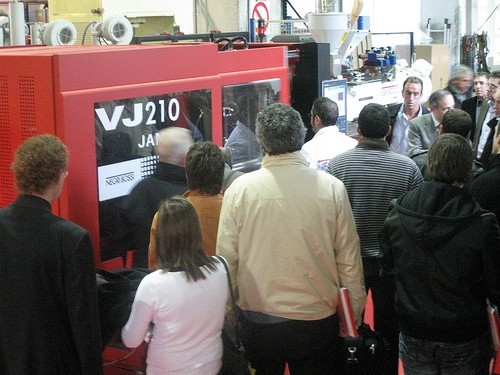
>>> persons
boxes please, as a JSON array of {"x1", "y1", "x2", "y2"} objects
[
  {"x1": 0, "y1": 66, "x2": 500, "y2": 375},
  {"x1": 225, "y1": 93, "x2": 267, "y2": 165}
]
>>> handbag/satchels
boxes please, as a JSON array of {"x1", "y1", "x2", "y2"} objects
[
  {"x1": 345, "y1": 322, "x2": 382, "y2": 375},
  {"x1": 220, "y1": 330, "x2": 250, "y2": 375}
]
